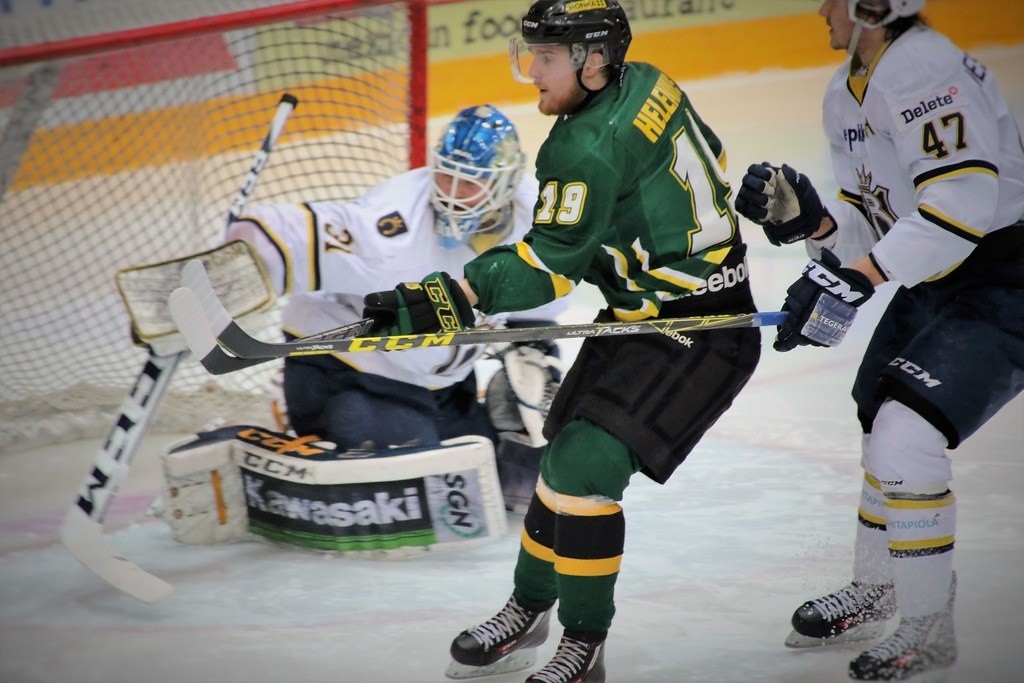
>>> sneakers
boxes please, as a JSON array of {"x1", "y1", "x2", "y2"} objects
[
  {"x1": 848, "y1": 614, "x2": 957, "y2": 682},
  {"x1": 784, "y1": 577, "x2": 896, "y2": 649},
  {"x1": 445, "y1": 594, "x2": 550, "y2": 679},
  {"x1": 523, "y1": 633, "x2": 607, "y2": 683}
]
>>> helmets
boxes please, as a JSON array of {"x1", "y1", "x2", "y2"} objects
[
  {"x1": 509, "y1": 0, "x2": 632, "y2": 83},
  {"x1": 846, "y1": 0, "x2": 925, "y2": 59},
  {"x1": 429, "y1": 103, "x2": 523, "y2": 241}
]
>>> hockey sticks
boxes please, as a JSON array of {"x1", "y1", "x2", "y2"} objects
[
  {"x1": 60, "y1": 92, "x2": 304, "y2": 607},
  {"x1": 161, "y1": 284, "x2": 376, "y2": 379},
  {"x1": 176, "y1": 253, "x2": 790, "y2": 364}
]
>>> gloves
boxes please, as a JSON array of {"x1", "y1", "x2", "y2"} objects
[
  {"x1": 734, "y1": 160, "x2": 837, "y2": 246},
  {"x1": 362, "y1": 271, "x2": 475, "y2": 337},
  {"x1": 773, "y1": 249, "x2": 876, "y2": 353}
]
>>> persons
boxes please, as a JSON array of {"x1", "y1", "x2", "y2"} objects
[
  {"x1": 225, "y1": 1, "x2": 761, "y2": 683},
  {"x1": 736, "y1": 0, "x2": 1024, "y2": 681}
]
